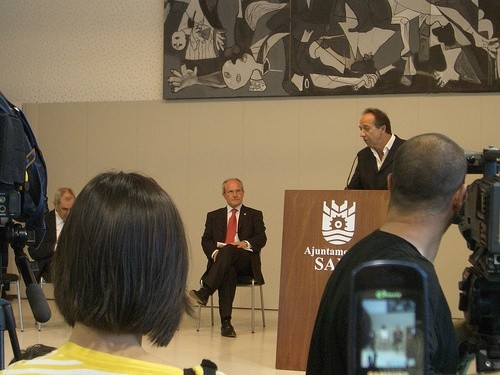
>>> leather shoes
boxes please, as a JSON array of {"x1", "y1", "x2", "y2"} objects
[
  {"x1": 222, "y1": 324, "x2": 236, "y2": 336},
  {"x1": 190, "y1": 288, "x2": 208, "y2": 308}
]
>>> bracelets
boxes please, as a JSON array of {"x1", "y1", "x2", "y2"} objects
[{"x1": 244, "y1": 242, "x2": 248, "y2": 248}]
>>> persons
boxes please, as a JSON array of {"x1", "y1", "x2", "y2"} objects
[
  {"x1": 379, "y1": 321, "x2": 404, "y2": 352},
  {"x1": 344, "y1": 108, "x2": 407, "y2": 191},
  {"x1": 41, "y1": 187, "x2": 76, "y2": 283},
  {"x1": 0, "y1": 168, "x2": 229, "y2": 375},
  {"x1": 189, "y1": 178, "x2": 268, "y2": 337},
  {"x1": 306, "y1": 132, "x2": 478, "y2": 375}
]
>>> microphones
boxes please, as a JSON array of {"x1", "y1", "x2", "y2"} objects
[
  {"x1": 345, "y1": 150, "x2": 366, "y2": 190},
  {"x1": 14, "y1": 246, "x2": 51, "y2": 323}
]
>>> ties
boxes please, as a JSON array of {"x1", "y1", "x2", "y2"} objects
[{"x1": 226, "y1": 208, "x2": 238, "y2": 243}]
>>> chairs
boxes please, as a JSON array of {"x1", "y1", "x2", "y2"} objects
[{"x1": 196, "y1": 271, "x2": 266, "y2": 333}]
